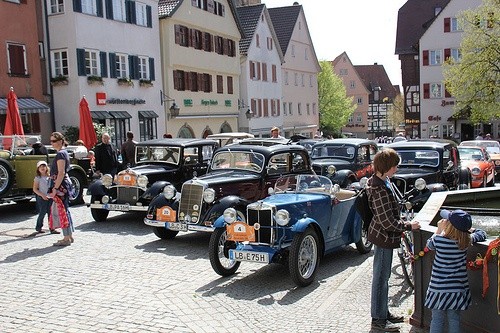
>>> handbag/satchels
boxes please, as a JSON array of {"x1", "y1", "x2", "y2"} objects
[{"x1": 355, "y1": 187, "x2": 373, "y2": 232}]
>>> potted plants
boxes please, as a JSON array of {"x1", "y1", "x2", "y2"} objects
[
  {"x1": 140, "y1": 79, "x2": 154, "y2": 87},
  {"x1": 118, "y1": 78, "x2": 135, "y2": 88},
  {"x1": 88, "y1": 75, "x2": 105, "y2": 86},
  {"x1": 51, "y1": 74, "x2": 69, "y2": 86}
]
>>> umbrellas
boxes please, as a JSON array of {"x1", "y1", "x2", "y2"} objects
[
  {"x1": 4, "y1": 86, "x2": 27, "y2": 149},
  {"x1": 78, "y1": 97, "x2": 97, "y2": 152}
]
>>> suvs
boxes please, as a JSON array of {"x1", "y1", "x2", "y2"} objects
[{"x1": 0, "y1": 132, "x2": 92, "y2": 207}]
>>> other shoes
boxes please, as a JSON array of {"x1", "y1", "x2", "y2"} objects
[
  {"x1": 53, "y1": 238, "x2": 74, "y2": 246},
  {"x1": 386, "y1": 312, "x2": 404, "y2": 322},
  {"x1": 51, "y1": 231, "x2": 60, "y2": 234},
  {"x1": 371, "y1": 317, "x2": 401, "y2": 332},
  {"x1": 39, "y1": 230, "x2": 45, "y2": 233}
]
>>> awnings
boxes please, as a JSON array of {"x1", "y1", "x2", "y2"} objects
[{"x1": 0, "y1": 98, "x2": 51, "y2": 115}]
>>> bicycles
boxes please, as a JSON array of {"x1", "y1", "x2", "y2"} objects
[{"x1": 393, "y1": 177, "x2": 430, "y2": 290}]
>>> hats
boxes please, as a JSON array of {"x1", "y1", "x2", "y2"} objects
[
  {"x1": 37, "y1": 161, "x2": 47, "y2": 168},
  {"x1": 440, "y1": 209, "x2": 472, "y2": 233}
]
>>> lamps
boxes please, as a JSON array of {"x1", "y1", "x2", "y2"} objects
[
  {"x1": 238, "y1": 98, "x2": 251, "y2": 120},
  {"x1": 161, "y1": 89, "x2": 180, "y2": 118}
]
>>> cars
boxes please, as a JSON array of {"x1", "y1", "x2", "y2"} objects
[
  {"x1": 308, "y1": 137, "x2": 380, "y2": 190},
  {"x1": 442, "y1": 145, "x2": 496, "y2": 188},
  {"x1": 458, "y1": 140, "x2": 500, "y2": 182},
  {"x1": 383, "y1": 139, "x2": 473, "y2": 214},
  {"x1": 142, "y1": 139, "x2": 312, "y2": 242},
  {"x1": 207, "y1": 168, "x2": 374, "y2": 288},
  {"x1": 83, "y1": 137, "x2": 220, "y2": 223},
  {"x1": 200, "y1": 133, "x2": 460, "y2": 178}
]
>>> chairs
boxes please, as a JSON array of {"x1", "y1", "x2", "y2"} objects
[
  {"x1": 334, "y1": 148, "x2": 346, "y2": 157},
  {"x1": 472, "y1": 154, "x2": 482, "y2": 160},
  {"x1": 23, "y1": 141, "x2": 46, "y2": 155}
]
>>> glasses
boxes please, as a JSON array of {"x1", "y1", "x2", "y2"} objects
[{"x1": 50, "y1": 138, "x2": 62, "y2": 143}]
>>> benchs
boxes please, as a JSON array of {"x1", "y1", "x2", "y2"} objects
[{"x1": 301, "y1": 185, "x2": 357, "y2": 200}]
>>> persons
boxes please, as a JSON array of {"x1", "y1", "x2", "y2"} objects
[
  {"x1": 49, "y1": 131, "x2": 75, "y2": 247},
  {"x1": 365, "y1": 146, "x2": 420, "y2": 332},
  {"x1": 474, "y1": 131, "x2": 492, "y2": 140},
  {"x1": 32, "y1": 160, "x2": 60, "y2": 234},
  {"x1": 424, "y1": 208, "x2": 487, "y2": 333},
  {"x1": 121, "y1": 131, "x2": 140, "y2": 168},
  {"x1": 270, "y1": 126, "x2": 286, "y2": 139},
  {"x1": 92, "y1": 133, "x2": 115, "y2": 180}
]
